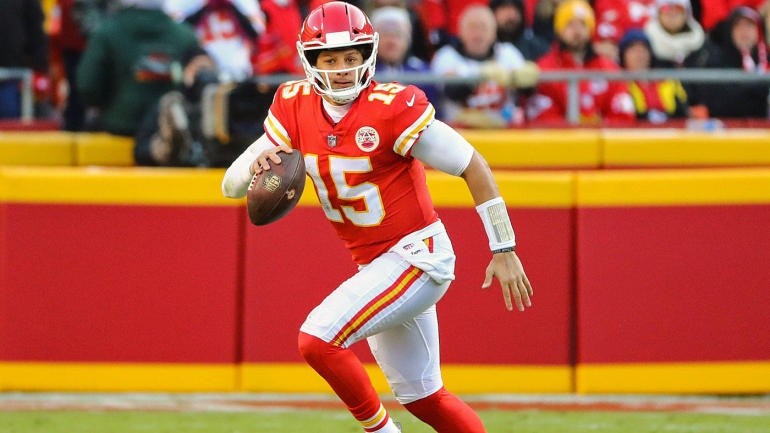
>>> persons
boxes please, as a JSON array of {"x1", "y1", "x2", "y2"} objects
[
  {"x1": 0, "y1": 0, "x2": 769, "y2": 171},
  {"x1": 220, "y1": 0, "x2": 536, "y2": 433}
]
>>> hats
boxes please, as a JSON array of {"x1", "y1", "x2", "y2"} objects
[
  {"x1": 554, "y1": 1, "x2": 596, "y2": 36},
  {"x1": 655, "y1": 0, "x2": 691, "y2": 21},
  {"x1": 619, "y1": 28, "x2": 652, "y2": 55},
  {"x1": 729, "y1": 6, "x2": 761, "y2": 24}
]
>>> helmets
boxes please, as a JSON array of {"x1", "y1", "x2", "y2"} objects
[{"x1": 297, "y1": 1, "x2": 378, "y2": 105}]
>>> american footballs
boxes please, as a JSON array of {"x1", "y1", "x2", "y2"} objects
[{"x1": 247, "y1": 148, "x2": 306, "y2": 225}]
